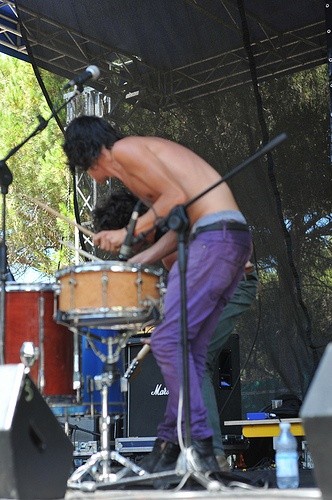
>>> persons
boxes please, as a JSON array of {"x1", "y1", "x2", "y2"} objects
[
  {"x1": 139, "y1": 211, "x2": 258, "y2": 470},
  {"x1": 63, "y1": 116, "x2": 253, "y2": 474}
]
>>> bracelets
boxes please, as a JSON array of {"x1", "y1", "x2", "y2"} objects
[{"x1": 125, "y1": 225, "x2": 136, "y2": 238}]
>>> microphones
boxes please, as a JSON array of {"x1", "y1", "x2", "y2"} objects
[
  {"x1": 119, "y1": 203, "x2": 141, "y2": 262},
  {"x1": 62, "y1": 65, "x2": 100, "y2": 90}
]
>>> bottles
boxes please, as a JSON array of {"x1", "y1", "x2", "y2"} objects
[{"x1": 275, "y1": 423, "x2": 298, "y2": 489}]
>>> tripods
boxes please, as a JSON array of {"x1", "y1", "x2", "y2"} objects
[{"x1": 67, "y1": 131, "x2": 288, "y2": 492}]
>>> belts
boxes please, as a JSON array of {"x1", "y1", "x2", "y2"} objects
[
  {"x1": 191, "y1": 223, "x2": 250, "y2": 242},
  {"x1": 240, "y1": 274, "x2": 258, "y2": 282}
]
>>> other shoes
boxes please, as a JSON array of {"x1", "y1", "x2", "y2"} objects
[{"x1": 98, "y1": 436, "x2": 221, "y2": 490}]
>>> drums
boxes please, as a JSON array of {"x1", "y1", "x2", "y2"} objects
[
  {"x1": 0, "y1": 280, "x2": 85, "y2": 409},
  {"x1": 52, "y1": 261, "x2": 163, "y2": 328}
]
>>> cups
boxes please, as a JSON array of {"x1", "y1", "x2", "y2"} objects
[{"x1": 272, "y1": 400, "x2": 282, "y2": 409}]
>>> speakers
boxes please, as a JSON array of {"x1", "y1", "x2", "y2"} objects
[
  {"x1": 297, "y1": 342, "x2": 332, "y2": 500},
  {"x1": 125, "y1": 333, "x2": 243, "y2": 439},
  {"x1": 0, "y1": 361, "x2": 76, "y2": 500}
]
